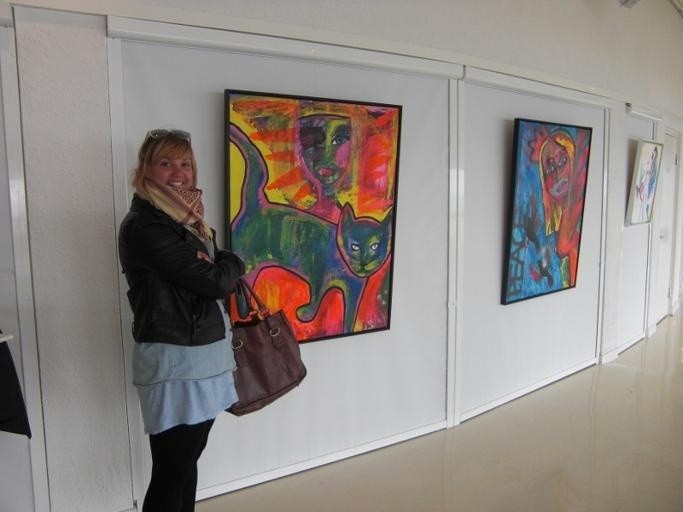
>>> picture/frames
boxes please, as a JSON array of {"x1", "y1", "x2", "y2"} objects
[
  {"x1": 621, "y1": 138, "x2": 664, "y2": 226},
  {"x1": 497, "y1": 114, "x2": 595, "y2": 306},
  {"x1": 220, "y1": 86, "x2": 405, "y2": 344}
]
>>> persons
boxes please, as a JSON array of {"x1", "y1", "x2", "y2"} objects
[
  {"x1": 540, "y1": 129, "x2": 588, "y2": 288},
  {"x1": 118, "y1": 129, "x2": 245, "y2": 512},
  {"x1": 240, "y1": 97, "x2": 397, "y2": 342},
  {"x1": 637, "y1": 146, "x2": 658, "y2": 222}
]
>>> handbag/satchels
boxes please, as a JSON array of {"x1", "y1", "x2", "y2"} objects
[{"x1": 223, "y1": 309, "x2": 306, "y2": 416}]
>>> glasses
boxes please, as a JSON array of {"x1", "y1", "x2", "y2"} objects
[{"x1": 143, "y1": 129, "x2": 192, "y2": 146}]
